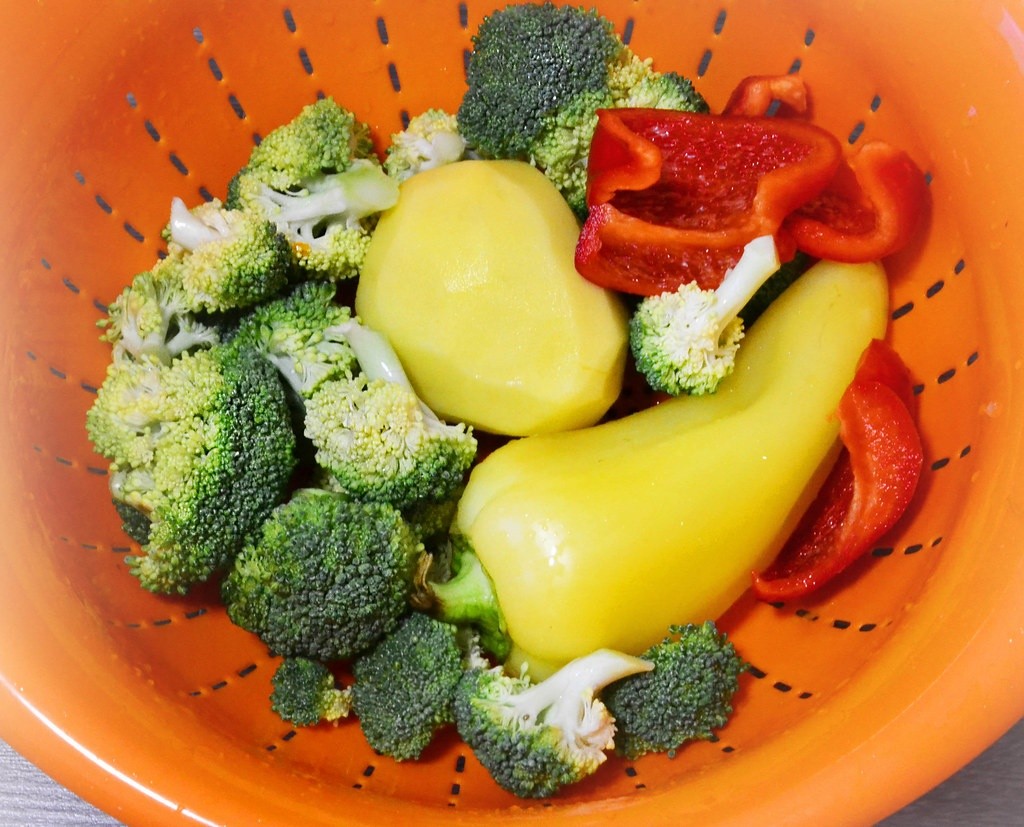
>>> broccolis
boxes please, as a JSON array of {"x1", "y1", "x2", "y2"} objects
[{"x1": 85, "y1": 2, "x2": 780, "y2": 801}]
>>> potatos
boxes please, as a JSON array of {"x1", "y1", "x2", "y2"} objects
[{"x1": 351, "y1": 160, "x2": 627, "y2": 437}]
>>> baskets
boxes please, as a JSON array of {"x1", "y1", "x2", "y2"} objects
[{"x1": 0, "y1": 0, "x2": 1024, "y2": 827}]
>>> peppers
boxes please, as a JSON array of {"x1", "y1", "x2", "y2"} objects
[{"x1": 419, "y1": 70, "x2": 927, "y2": 687}]
